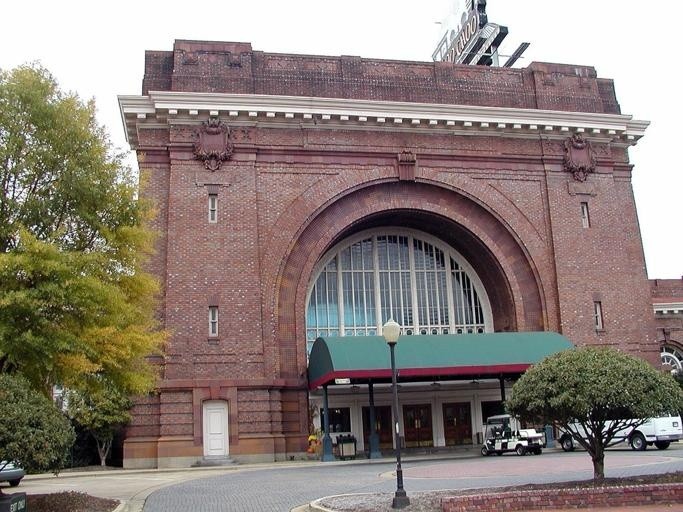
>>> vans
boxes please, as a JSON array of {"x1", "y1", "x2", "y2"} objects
[{"x1": 558, "y1": 404, "x2": 683, "y2": 452}]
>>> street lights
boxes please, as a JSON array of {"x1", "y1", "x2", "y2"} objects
[{"x1": 380, "y1": 316, "x2": 411, "y2": 508}]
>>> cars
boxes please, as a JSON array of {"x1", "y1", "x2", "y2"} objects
[{"x1": 0, "y1": 455, "x2": 24, "y2": 489}]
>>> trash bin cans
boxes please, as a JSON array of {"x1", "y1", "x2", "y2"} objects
[{"x1": 336, "y1": 434, "x2": 357, "y2": 460}]
